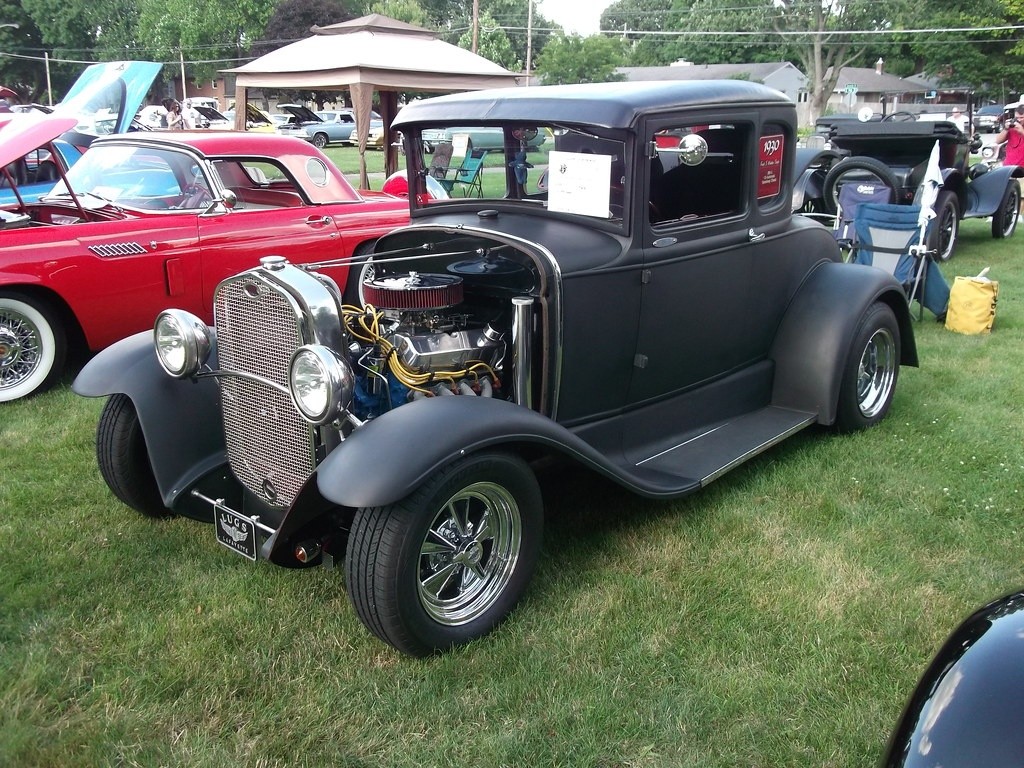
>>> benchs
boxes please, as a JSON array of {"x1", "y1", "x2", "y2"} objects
[
  {"x1": 829, "y1": 119, "x2": 969, "y2": 169},
  {"x1": 186, "y1": 186, "x2": 301, "y2": 218}
]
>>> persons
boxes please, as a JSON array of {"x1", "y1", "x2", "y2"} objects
[
  {"x1": 994, "y1": 104, "x2": 1024, "y2": 222},
  {"x1": 945, "y1": 107, "x2": 975, "y2": 136},
  {"x1": 165, "y1": 101, "x2": 183, "y2": 130}
]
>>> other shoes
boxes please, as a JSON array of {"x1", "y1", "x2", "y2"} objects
[
  {"x1": 1017, "y1": 214, "x2": 1023, "y2": 223},
  {"x1": 986, "y1": 216, "x2": 993, "y2": 223}
]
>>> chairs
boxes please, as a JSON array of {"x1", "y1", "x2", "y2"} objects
[
  {"x1": 562, "y1": 131, "x2": 664, "y2": 200},
  {"x1": 652, "y1": 128, "x2": 749, "y2": 220},
  {"x1": 839, "y1": 202, "x2": 933, "y2": 324},
  {"x1": 433, "y1": 148, "x2": 488, "y2": 199},
  {"x1": 426, "y1": 142, "x2": 454, "y2": 181},
  {"x1": 34, "y1": 160, "x2": 58, "y2": 181},
  {"x1": 796, "y1": 183, "x2": 891, "y2": 264},
  {"x1": 0, "y1": 156, "x2": 29, "y2": 188}
]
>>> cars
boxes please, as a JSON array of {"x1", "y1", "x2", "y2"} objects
[
  {"x1": 0, "y1": 133, "x2": 450, "y2": 406},
  {"x1": 347, "y1": 119, "x2": 384, "y2": 150},
  {"x1": 275, "y1": 104, "x2": 357, "y2": 149},
  {"x1": 789, "y1": 90, "x2": 1024, "y2": 263},
  {"x1": 0, "y1": 62, "x2": 264, "y2": 209},
  {"x1": 71, "y1": 80, "x2": 921, "y2": 658}
]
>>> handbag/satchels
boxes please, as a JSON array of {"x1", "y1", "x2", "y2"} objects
[{"x1": 944, "y1": 276, "x2": 999, "y2": 336}]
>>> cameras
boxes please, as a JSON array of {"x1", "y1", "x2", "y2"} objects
[{"x1": 1008, "y1": 118, "x2": 1016, "y2": 128}]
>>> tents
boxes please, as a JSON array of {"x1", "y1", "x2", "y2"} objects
[{"x1": 215, "y1": 12, "x2": 535, "y2": 200}]
